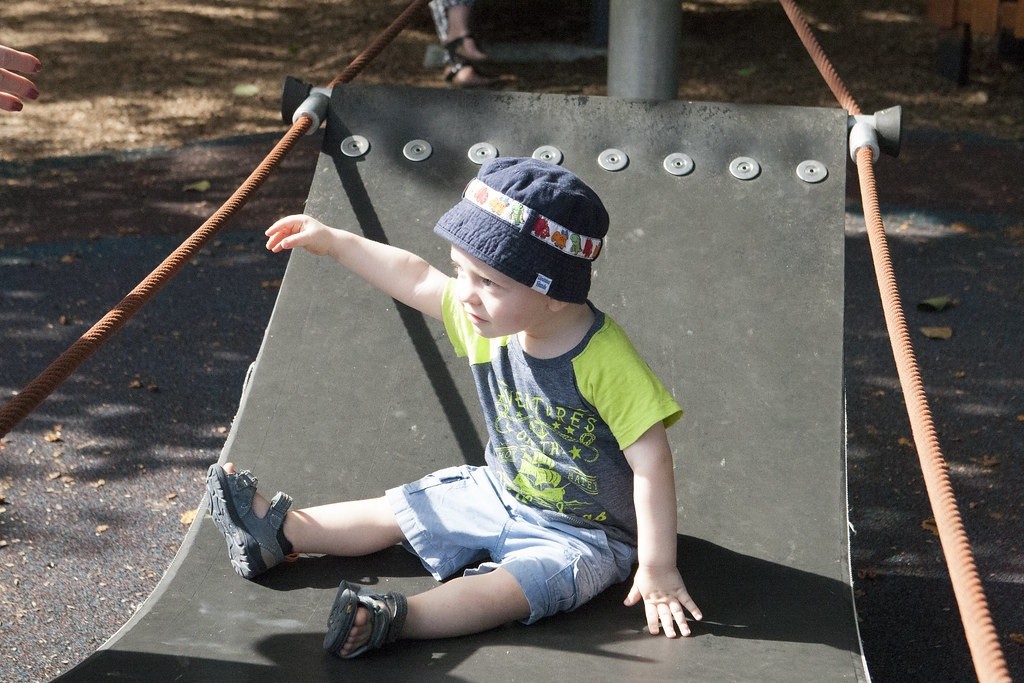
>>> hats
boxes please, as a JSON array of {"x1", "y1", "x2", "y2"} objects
[{"x1": 434, "y1": 156, "x2": 609, "y2": 306}]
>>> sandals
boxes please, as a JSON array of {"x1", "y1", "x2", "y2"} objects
[
  {"x1": 208, "y1": 463, "x2": 293, "y2": 578},
  {"x1": 323, "y1": 580, "x2": 408, "y2": 658}
]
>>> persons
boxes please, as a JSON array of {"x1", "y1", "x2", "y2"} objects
[{"x1": 206, "y1": 156, "x2": 703, "y2": 661}]
